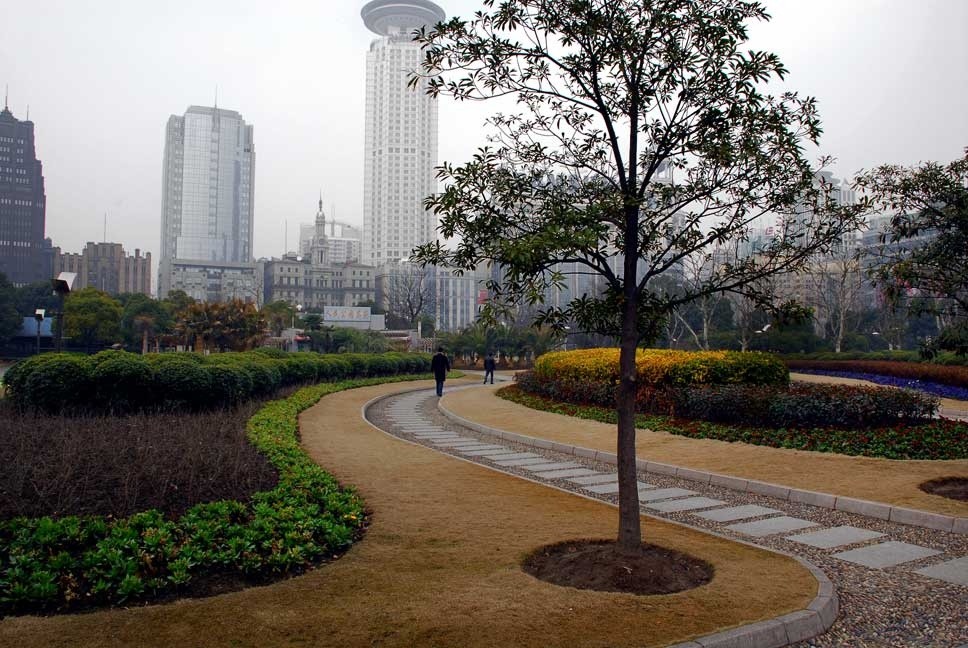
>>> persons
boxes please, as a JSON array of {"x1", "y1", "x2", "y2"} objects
[
  {"x1": 431, "y1": 347, "x2": 451, "y2": 396},
  {"x1": 484, "y1": 352, "x2": 496, "y2": 384}
]
